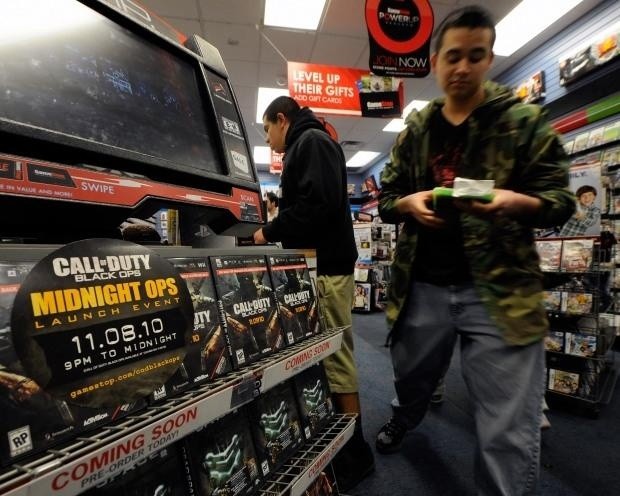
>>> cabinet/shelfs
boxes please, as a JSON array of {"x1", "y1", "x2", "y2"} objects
[
  {"x1": 1, "y1": 243, "x2": 359, "y2": 496},
  {"x1": 351, "y1": 91, "x2": 620, "y2": 419}
]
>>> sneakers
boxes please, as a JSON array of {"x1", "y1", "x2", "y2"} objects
[
  {"x1": 337, "y1": 442, "x2": 375, "y2": 493},
  {"x1": 430, "y1": 383, "x2": 446, "y2": 403},
  {"x1": 375, "y1": 418, "x2": 406, "y2": 455}
]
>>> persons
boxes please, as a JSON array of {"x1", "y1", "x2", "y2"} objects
[
  {"x1": 378, "y1": 6, "x2": 575, "y2": 453},
  {"x1": 254, "y1": 96, "x2": 372, "y2": 454},
  {"x1": 264, "y1": 192, "x2": 279, "y2": 222},
  {"x1": 561, "y1": 185, "x2": 601, "y2": 236}
]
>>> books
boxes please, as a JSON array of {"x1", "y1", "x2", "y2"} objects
[
  {"x1": 0, "y1": 255, "x2": 333, "y2": 496},
  {"x1": 535, "y1": 240, "x2": 619, "y2": 394},
  {"x1": 562, "y1": 122, "x2": 620, "y2": 239}
]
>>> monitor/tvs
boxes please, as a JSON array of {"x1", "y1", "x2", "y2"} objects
[{"x1": 0, "y1": 0, "x2": 228, "y2": 176}]
